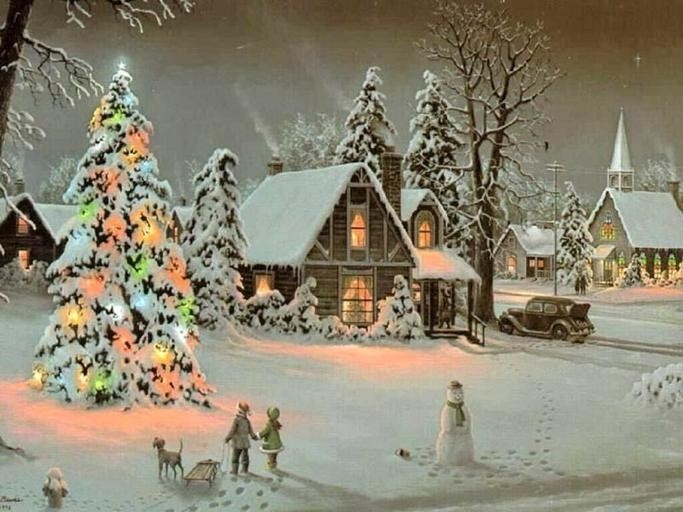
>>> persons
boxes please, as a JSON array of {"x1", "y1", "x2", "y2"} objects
[
  {"x1": 223, "y1": 400, "x2": 257, "y2": 474},
  {"x1": 437, "y1": 287, "x2": 452, "y2": 329},
  {"x1": 574, "y1": 276, "x2": 581, "y2": 295},
  {"x1": 579, "y1": 274, "x2": 588, "y2": 296},
  {"x1": 256, "y1": 404, "x2": 284, "y2": 473}
]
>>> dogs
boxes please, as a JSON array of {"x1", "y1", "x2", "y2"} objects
[
  {"x1": 151, "y1": 435, "x2": 184, "y2": 482},
  {"x1": 41, "y1": 465, "x2": 66, "y2": 508}
]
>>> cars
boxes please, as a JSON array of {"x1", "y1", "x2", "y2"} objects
[{"x1": 496, "y1": 296, "x2": 594, "y2": 342}]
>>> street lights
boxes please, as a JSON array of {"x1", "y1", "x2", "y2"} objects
[{"x1": 543, "y1": 160, "x2": 565, "y2": 296}]
